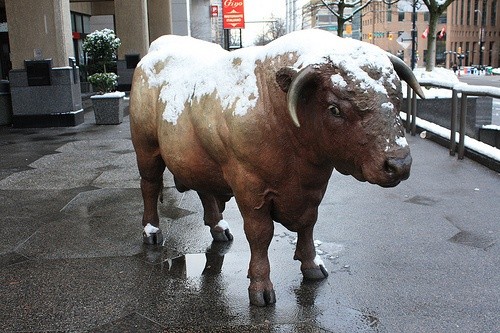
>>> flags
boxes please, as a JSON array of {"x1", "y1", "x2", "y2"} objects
[
  {"x1": 422, "y1": 26, "x2": 429, "y2": 38},
  {"x1": 439, "y1": 27, "x2": 446, "y2": 39}
]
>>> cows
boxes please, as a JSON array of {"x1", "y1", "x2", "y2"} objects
[{"x1": 129, "y1": 28, "x2": 427, "y2": 307}]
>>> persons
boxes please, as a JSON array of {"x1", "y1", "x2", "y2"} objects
[{"x1": 453, "y1": 63, "x2": 459, "y2": 74}]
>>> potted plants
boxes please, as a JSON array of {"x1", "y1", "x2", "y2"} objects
[{"x1": 81, "y1": 27, "x2": 125, "y2": 126}]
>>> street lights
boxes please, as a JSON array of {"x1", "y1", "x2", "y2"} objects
[
  {"x1": 457, "y1": 54, "x2": 465, "y2": 66},
  {"x1": 474, "y1": 10, "x2": 483, "y2": 67}
]
